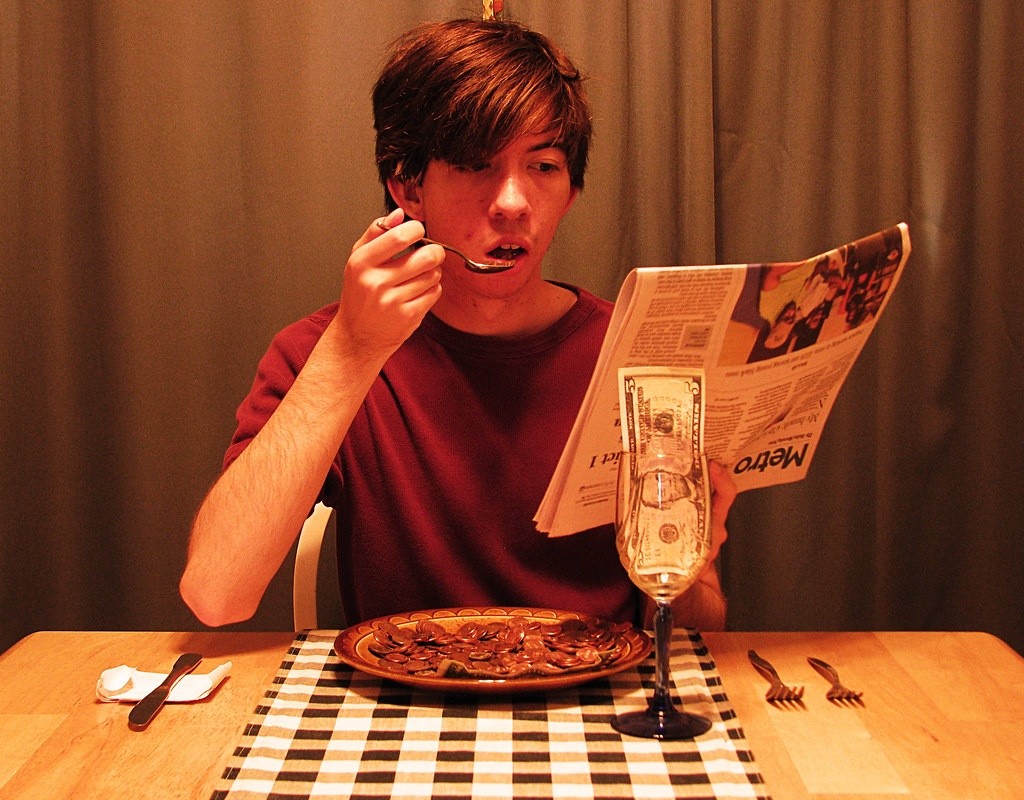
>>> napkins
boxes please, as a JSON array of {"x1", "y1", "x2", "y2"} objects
[{"x1": 96, "y1": 664, "x2": 231, "y2": 702}]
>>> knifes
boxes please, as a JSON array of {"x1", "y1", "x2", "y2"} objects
[{"x1": 128, "y1": 653, "x2": 202, "y2": 727}]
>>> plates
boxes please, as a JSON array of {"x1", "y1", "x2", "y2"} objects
[{"x1": 333, "y1": 605, "x2": 653, "y2": 703}]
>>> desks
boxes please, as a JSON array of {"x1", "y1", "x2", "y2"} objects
[{"x1": 0, "y1": 632, "x2": 1024, "y2": 800}]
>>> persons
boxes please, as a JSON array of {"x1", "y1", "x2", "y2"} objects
[
  {"x1": 757, "y1": 245, "x2": 832, "y2": 347},
  {"x1": 179, "y1": 16, "x2": 739, "y2": 636}
]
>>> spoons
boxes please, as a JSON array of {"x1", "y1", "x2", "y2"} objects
[{"x1": 375, "y1": 222, "x2": 517, "y2": 274}]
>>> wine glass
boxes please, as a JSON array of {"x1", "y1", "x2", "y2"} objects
[{"x1": 609, "y1": 448, "x2": 714, "y2": 740}]
